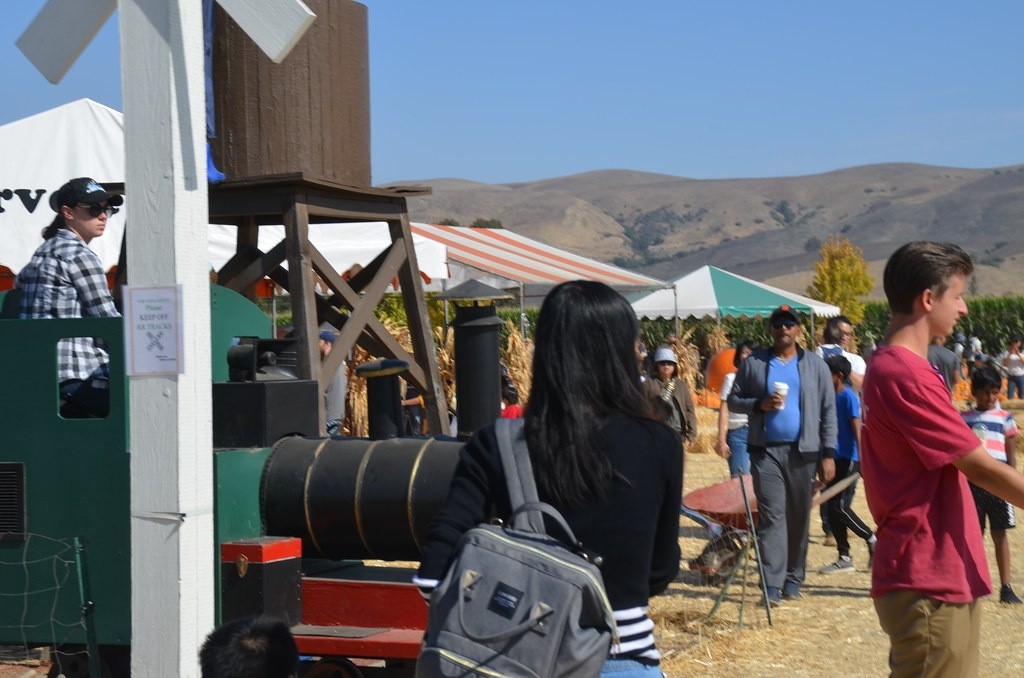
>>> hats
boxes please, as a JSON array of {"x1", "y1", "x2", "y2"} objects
[
  {"x1": 654, "y1": 348, "x2": 677, "y2": 365},
  {"x1": 57, "y1": 177, "x2": 123, "y2": 210},
  {"x1": 770, "y1": 305, "x2": 801, "y2": 323},
  {"x1": 321, "y1": 330, "x2": 336, "y2": 343}
]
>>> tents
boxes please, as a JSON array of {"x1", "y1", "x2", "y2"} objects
[
  {"x1": 0, "y1": 97, "x2": 678, "y2": 349},
  {"x1": 631, "y1": 266, "x2": 841, "y2": 351}
]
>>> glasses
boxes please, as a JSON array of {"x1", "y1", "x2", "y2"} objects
[
  {"x1": 70, "y1": 202, "x2": 113, "y2": 218},
  {"x1": 770, "y1": 318, "x2": 797, "y2": 328}
]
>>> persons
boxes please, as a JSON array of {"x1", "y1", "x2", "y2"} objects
[
  {"x1": 318, "y1": 332, "x2": 346, "y2": 437},
  {"x1": 13, "y1": 178, "x2": 123, "y2": 414},
  {"x1": 198, "y1": 615, "x2": 303, "y2": 678},
  {"x1": 415, "y1": 241, "x2": 1024, "y2": 678}
]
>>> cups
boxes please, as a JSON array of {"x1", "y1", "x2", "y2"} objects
[
  {"x1": 972, "y1": 423, "x2": 988, "y2": 451},
  {"x1": 772, "y1": 382, "x2": 790, "y2": 410}
]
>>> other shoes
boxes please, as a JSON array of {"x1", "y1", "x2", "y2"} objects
[
  {"x1": 820, "y1": 556, "x2": 854, "y2": 574},
  {"x1": 1000, "y1": 587, "x2": 1021, "y2": 603},
  {"x1": 826, "y1": 535, "x2": 836, "y2": 546},
  {"x1": 867, "y1": 540, "x2": 877, "y2": 565}
]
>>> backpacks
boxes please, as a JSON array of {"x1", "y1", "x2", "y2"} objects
[{"x1": 413, "y1": 418, "x2": 619, "y2": 678}]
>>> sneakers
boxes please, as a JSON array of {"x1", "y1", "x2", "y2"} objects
[
  {"x1": 761, "y1": 587, "x2": 780, "y2": 607},
  {"x1": 783, "y1": 579, "x2": 801, "y2": 600}
]
